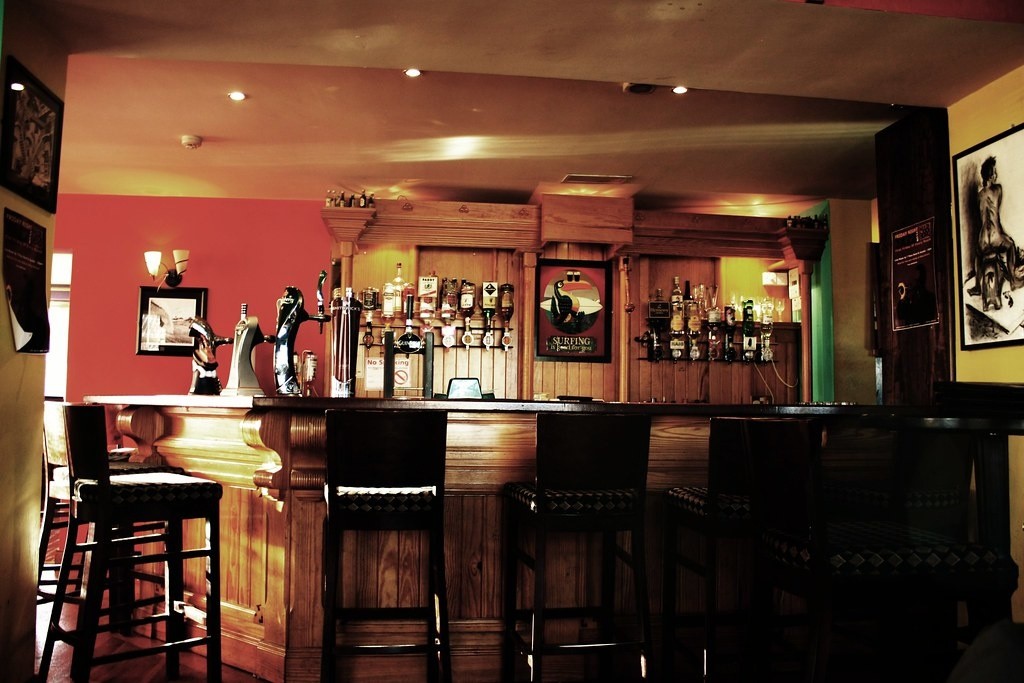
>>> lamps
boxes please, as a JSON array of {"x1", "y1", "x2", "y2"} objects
[{"x1": 143, "y1": 249, "x2": 190, "y2": 288}]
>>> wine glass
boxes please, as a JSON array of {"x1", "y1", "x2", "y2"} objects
[{"x1": 729, "y1": 293, "x2": 785, "y2": 322}]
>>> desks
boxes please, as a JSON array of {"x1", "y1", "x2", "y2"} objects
[{"x1": 84, "y1": 393, "x2": 1015, "y2": 681}]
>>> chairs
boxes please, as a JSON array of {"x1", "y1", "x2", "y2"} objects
[
  {"x1": 658, "y1": 415, "x2": 817, "y2": 683},
  {"x1": 498, "y1": 412, "x2": 653, "y2": 683},
  {"x1": 39, "y1": 399, "x2": 188, "y2": 653},
  {"x1": 37, "y1": 404, "x2": 223, "y2": 683},
  {"x1": 744, "y1": 419, "x2": 1018, "y2": 682},
  {"x1": 319, "y1": 407, "x2": 452, "y2": 682}
]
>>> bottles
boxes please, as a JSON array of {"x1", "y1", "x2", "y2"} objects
[
  {"x1": 236, "y1": 302, "x2": 248, "y2": 335},
  {"x1": 294, "y1": 352, "x2": 318, "y2": 397},
  {"x1": 784, "y1": 215, "x2": 828, "y2": 230},
  {"x1": 326, "y1": 189, "x2": 375, "y2": 208},
  {"x1": 333, "y1": 262, "x2": 515, "y2": 352},
  {"x1": 646, "y1": 276, "x2": 774, "y2": 365}
]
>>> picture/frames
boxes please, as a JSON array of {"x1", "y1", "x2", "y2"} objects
[
  {"x1": 0, "y1": 53, "x2": 64, "y2": 215},
  {"x1": 952, "y1": 122, "x2": 1024, "y2": 352},
  {"x1": 135, "y1": 285, "x2": 209, "y2": 357}
]
